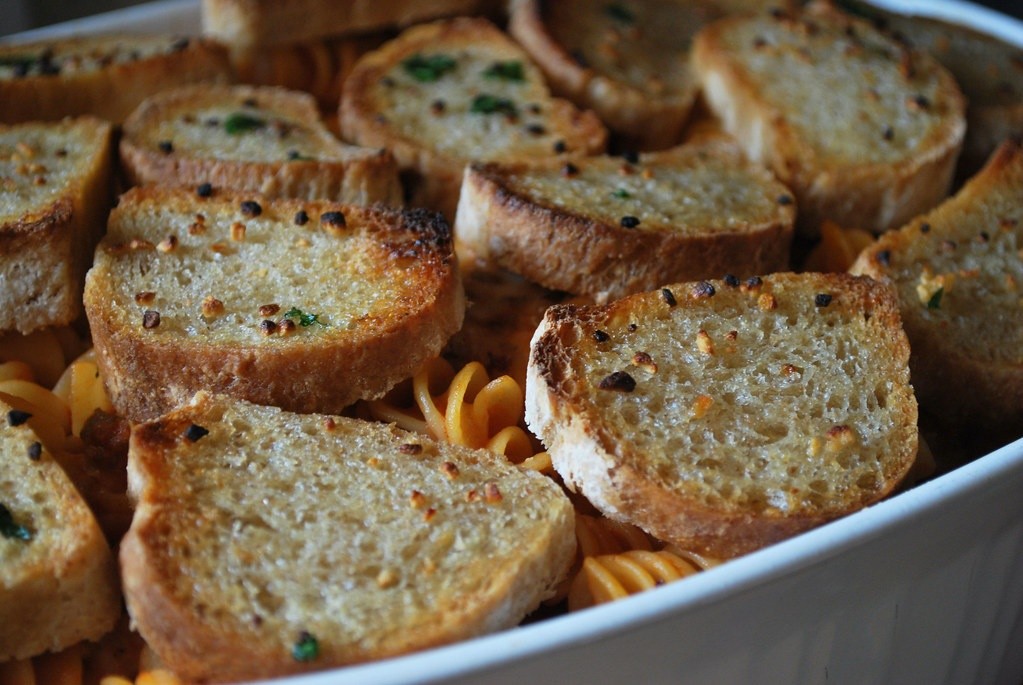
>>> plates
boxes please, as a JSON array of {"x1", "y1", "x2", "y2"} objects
[{"x1": 0, "y1": 0, "x2": 1023, "y2": 685}]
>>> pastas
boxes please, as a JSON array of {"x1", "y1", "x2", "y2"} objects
[{"x1": 0, "y1": 219, "x2": 957, "y2": 685}]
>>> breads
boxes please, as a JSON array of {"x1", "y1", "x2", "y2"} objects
[{"x1": 0, "y1": 0, "x2": 1023, "y2": 680}]
